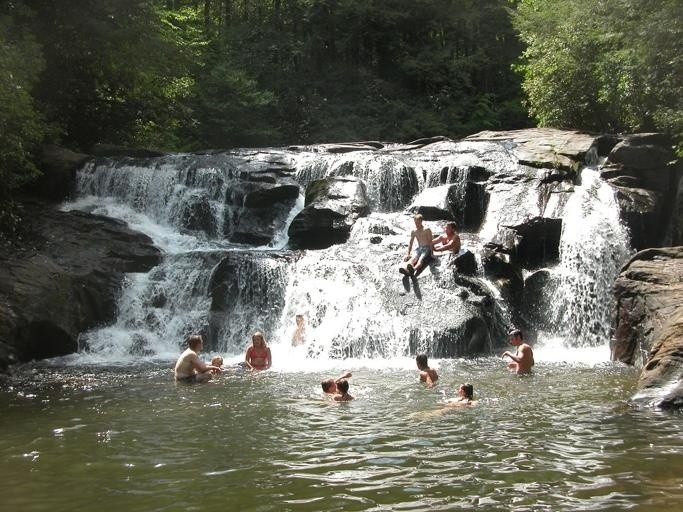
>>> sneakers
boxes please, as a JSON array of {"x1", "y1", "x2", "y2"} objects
[
  {"x1": 407, "y1": 264, "x2": 414, "y2": 274},
  {"x1": 399, "y1": 268, "x2": 409, "y2": 276}
]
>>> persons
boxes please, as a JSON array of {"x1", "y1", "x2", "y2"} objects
[
  {"x1": 501, "y1": 329, "x2": 534, "y2": 375},
  {"x1": 398, "y1": 213, "x2": 432, "y2": 277},
  {"x1": 320, "y1": 370, "x2": 354, "y2": 396},
  {"x1": 291, "y1": 314, "x2": 306, "y2": 346},
  {"x1": 431, "y1": 221, "x2": 460, "y2": 252},
  {"x1": 415, "y1": 355, "x2": 438, "y2": 383},
  {"x1": 173, "y1": 334, "x2": 222, "y2": 384},
  {"x1": 210, "y1": 355, "x2": 225, "y2": 371},
  {"x1": 406, "y1": 383, "x2": 477, "y2": 423},
  {"x1": 333, "y1": 378, "x2": 353, "y2": 401},
  {"x1": 245, "y1": 332, "x2": 271, "y2": 372}
]
